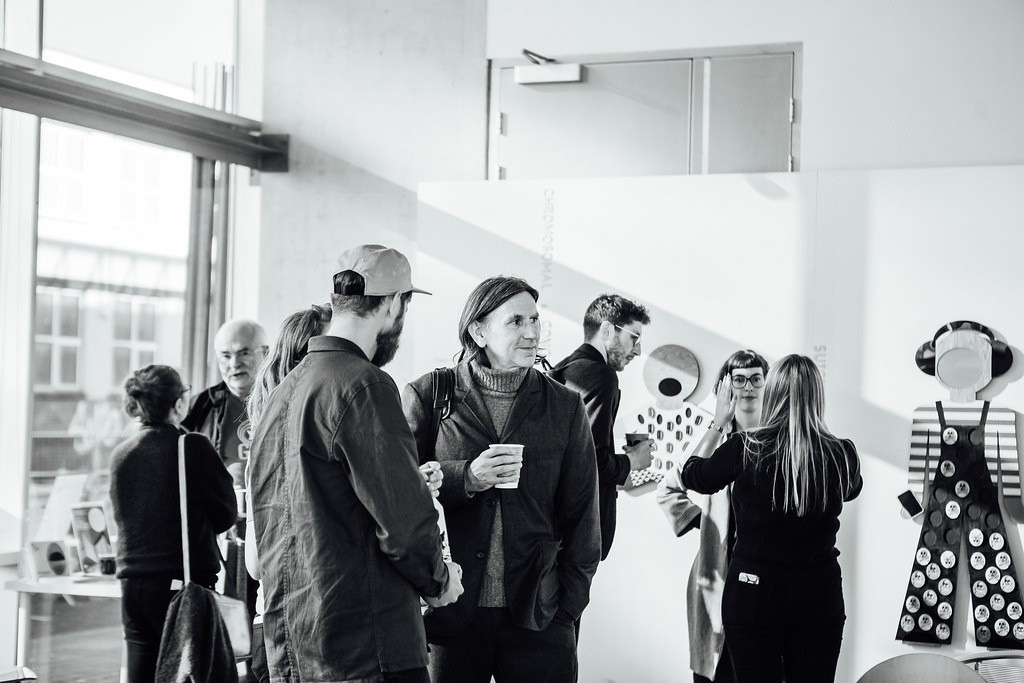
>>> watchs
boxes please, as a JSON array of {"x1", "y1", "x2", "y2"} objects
[{"x1": 708, "y1": 420, "x2": 723, "y2": 434}]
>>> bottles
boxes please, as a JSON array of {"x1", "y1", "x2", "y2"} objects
[{"x1": 423, "y1": 468, "x2": 452, "y2": 562}]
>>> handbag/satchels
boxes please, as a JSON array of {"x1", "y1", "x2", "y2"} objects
[{"x1": 185, "y1": 583, "x2": 253, "y2": 683}]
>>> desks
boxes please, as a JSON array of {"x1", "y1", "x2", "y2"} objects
[{"x1": 3, "y1": 576, "x2": 128, "y2": 683}]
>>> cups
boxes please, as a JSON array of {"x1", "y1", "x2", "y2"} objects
[
  {"x1": 626, "y1": 434, "x2": 649, "y2": 471},
  {"x1": 489, "y1": 444, "x2": 524, "y2": 489},
  {"x1": 98, "y1": 554, "x2": 116, "y2": 574},
  {"x1": 235, "y1": 490, "x2": 246, "y2": 518}
]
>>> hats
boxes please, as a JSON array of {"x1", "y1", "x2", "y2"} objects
[{"x1": 331, "y1": 244, "x2": 433, "y2": 296}]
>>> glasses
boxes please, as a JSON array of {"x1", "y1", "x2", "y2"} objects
[
  {"x1": 172, "y1": 384, "x2": 192, "y2": 408},
  {"x1": 614, "y1": 325, "x2": 641, "y2": 347},
  {"x1": 217, "y1": 349, "x2": 263, "y2": 364},
  {"x1": 731, "y1": 373, "x2": 765, "y2": 389}
]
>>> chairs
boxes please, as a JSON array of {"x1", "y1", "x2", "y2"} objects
[
  {"x1": 857, "y1": 653, "x2": 987, "y2": 683},
  {"x1": 962, "y1": 654, "x2": 1024, "y2": 683}
]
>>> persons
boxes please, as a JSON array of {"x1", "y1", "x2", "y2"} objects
[
  {"x1": 657, "y1": 349, "x2": 864, "y2": 683},
  {"x1": 400, "y1": 277, "x2": 655, "y2": 682},
  {"x1": 109, "y1": 245, "x2": 465, "y2": 683}
]
